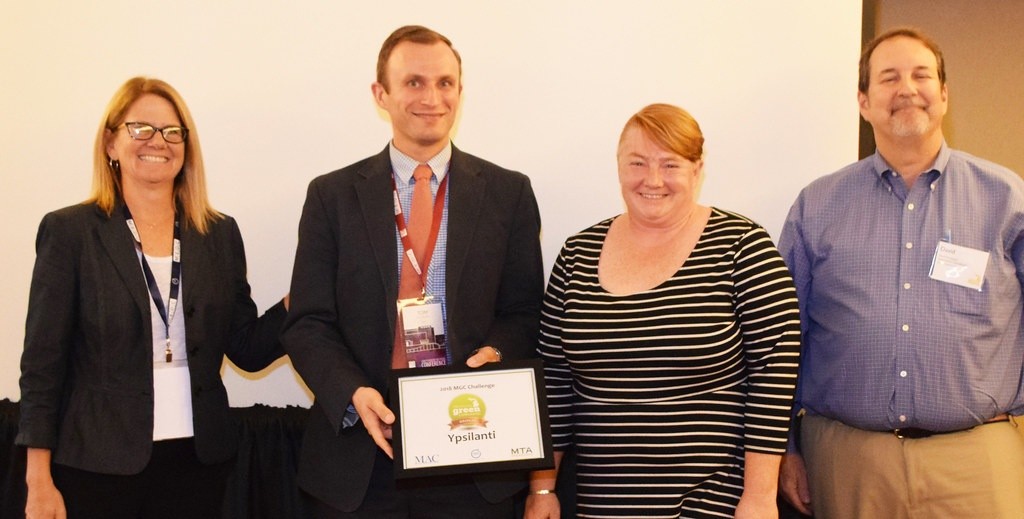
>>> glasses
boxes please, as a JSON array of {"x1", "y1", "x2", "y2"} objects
[{"x1": 112, "y1": 121, "x2": 189, "y2": 144}]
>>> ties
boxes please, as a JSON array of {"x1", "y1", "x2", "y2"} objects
[{"x1": 391, "y1": 164, "x2": 434, "y2": 369}]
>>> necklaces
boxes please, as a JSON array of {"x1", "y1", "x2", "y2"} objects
[{"x1": 142, "y1": 218, "x2": 161, "y2": 230}]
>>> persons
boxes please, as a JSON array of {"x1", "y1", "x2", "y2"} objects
[
  {"x1": 776, "y1": 27, "x2": 1024, "y2": 519},
  {"x1": 16, "y1": 77, "x2": 289, "y2": 519},
  {"x1": 279, "y1": 25, "x2": 543, "y2": 519},
  {"x1": 523, "y1": 103, "x2": 802, "y2": 519}
]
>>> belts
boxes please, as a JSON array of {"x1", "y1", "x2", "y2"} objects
[{"x1": 871, "y1": 414, "x2": 1010, "y2": 441}]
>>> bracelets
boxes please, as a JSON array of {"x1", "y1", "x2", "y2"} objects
[{"x1": 527, "y1": 489, "x2": 555, "y2": 497}]
